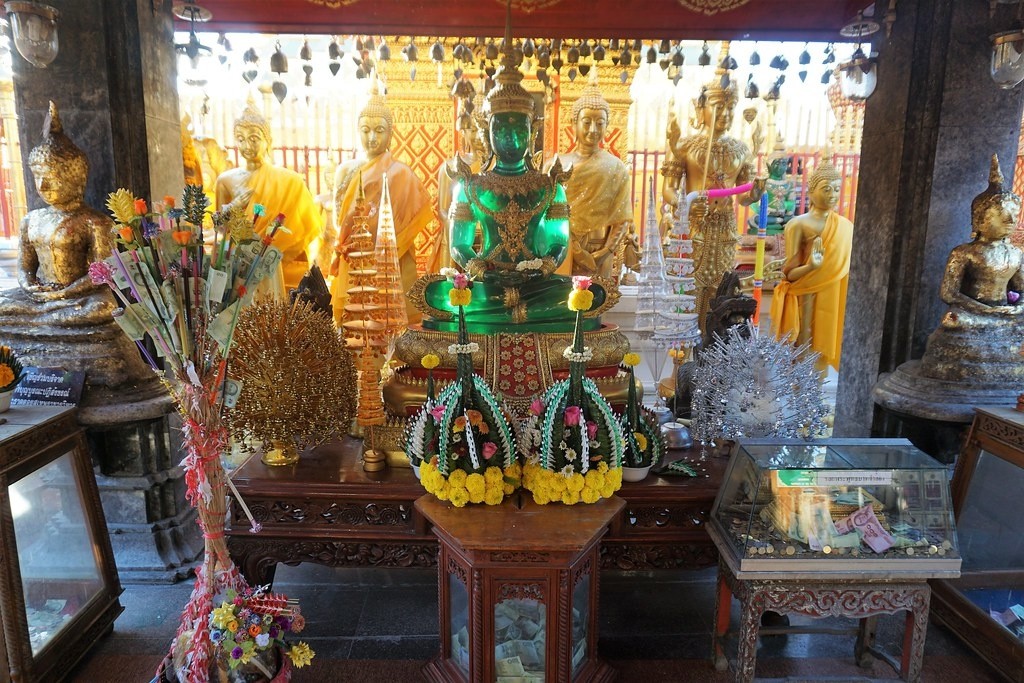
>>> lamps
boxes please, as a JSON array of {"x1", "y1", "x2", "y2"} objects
[
  {"x1": 988, "y1": 29, "x2": 1024, "y2": 90},
  {"x1": 2, "y1": 0, "x2": 61, "y2": 71},
  {"x1": 837, "y1": 10, "x2": 881, "y2": 101},
  {"x1": 171, "y1": 0, "x2": 214, "y2": 69}
]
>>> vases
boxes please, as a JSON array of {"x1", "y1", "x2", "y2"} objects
[
  {"x1": 155, "y1": 642, "x2": 294, "y2": 683},
  {"x1": 0, "y1": 389, "x2": 15, "y2": 413},
  {"x1": 621, "y1": 465, "x2": 652, "y2": 482}
]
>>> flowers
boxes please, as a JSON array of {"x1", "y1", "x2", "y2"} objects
[
  {"x1": 92, "y1": 184, "x2": 315, "y2": 683},
  {"x1": 615, "y1": 352, "x2": 660, "y2": 467},
  {"x1": 0, "y1": 345, "x2": 28, "y2": 393}
]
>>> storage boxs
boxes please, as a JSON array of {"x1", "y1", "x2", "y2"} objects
[
  {"x1": 925, "y1": 407, "x2": 1024, "y2": 683},
  {"x1": 703, "y1": 437, "x2": 962, "y2": 577},
  {"x1": 0, "y1": 403, "x2": 126, "y2": 683}
]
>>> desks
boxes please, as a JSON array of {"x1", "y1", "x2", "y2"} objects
[{"x1": 229, "y1": 430, "x2": 794, "y2": 643}]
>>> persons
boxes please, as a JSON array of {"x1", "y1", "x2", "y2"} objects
[
  {"x1": 541, "y1": 59, "x2": 634, "y2": 284},
  {"x1": 406, "y1": 0, "x2": 606, "y2": 333},
  {"x1": 624, "y1": 223, "x2": 641, "y2": 270},
  {"x1": 747, "y1": 129, "x2": 798, "y2": 237},
  {"x1": 659, "y1": 40, "x2": 772, "y2": 361},
  {"x1": 919, "y1": 152, "x2": 1022, "y2": 382},
  {"x1": 769, "y1": 143, "x2": 853, "y2": 426},
  {"x1": 436, "y1": 92, "x2": 497, "y2": 276},
  {"x1": 214, "y1": 90, "x2": 325, "y2": 300},
  {"x1": 330, "y1": 79, "x2": 437, "y2": 327},
  {"x1": 0, "y1": 99, "x2": 122, "y2": 326}
]
